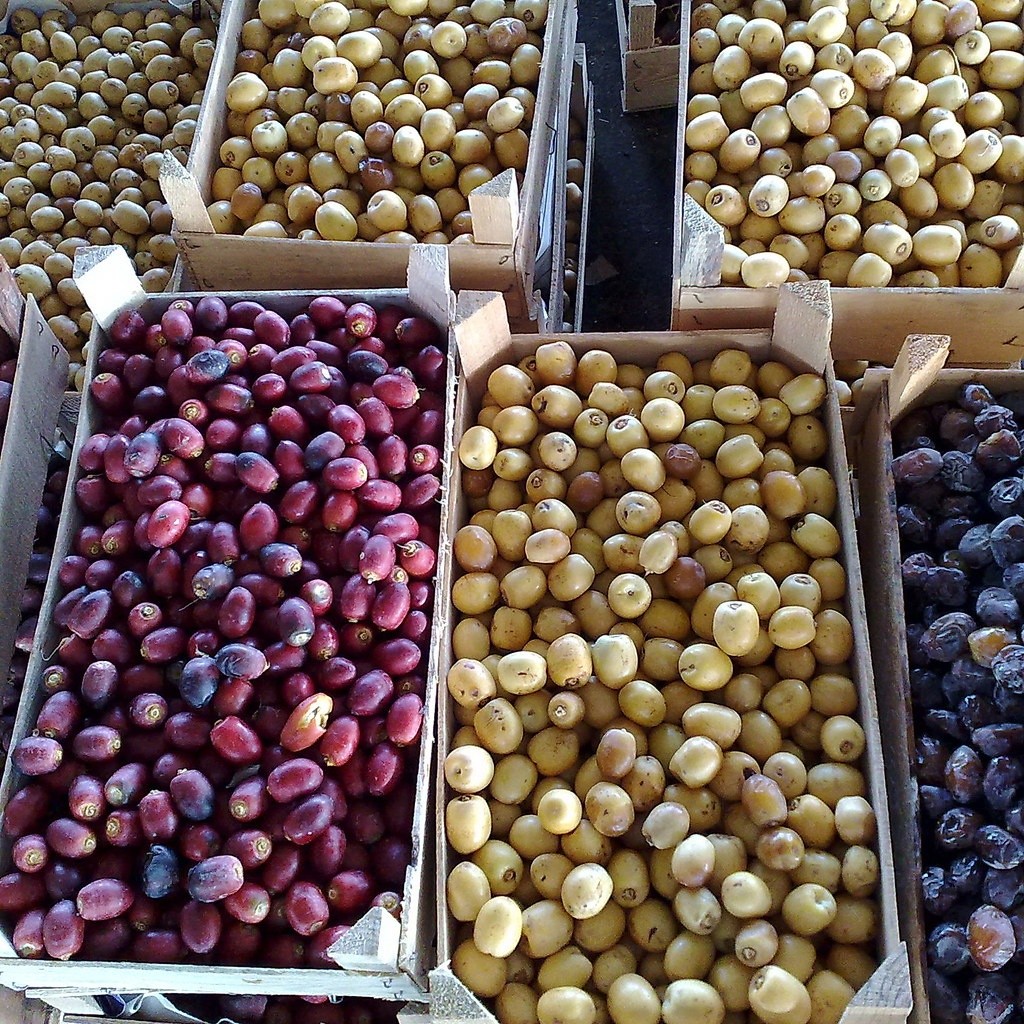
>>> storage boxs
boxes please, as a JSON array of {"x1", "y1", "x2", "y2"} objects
[{"x1": 0, "y1": 0, "x2": 1024, "y2": 1024}]
